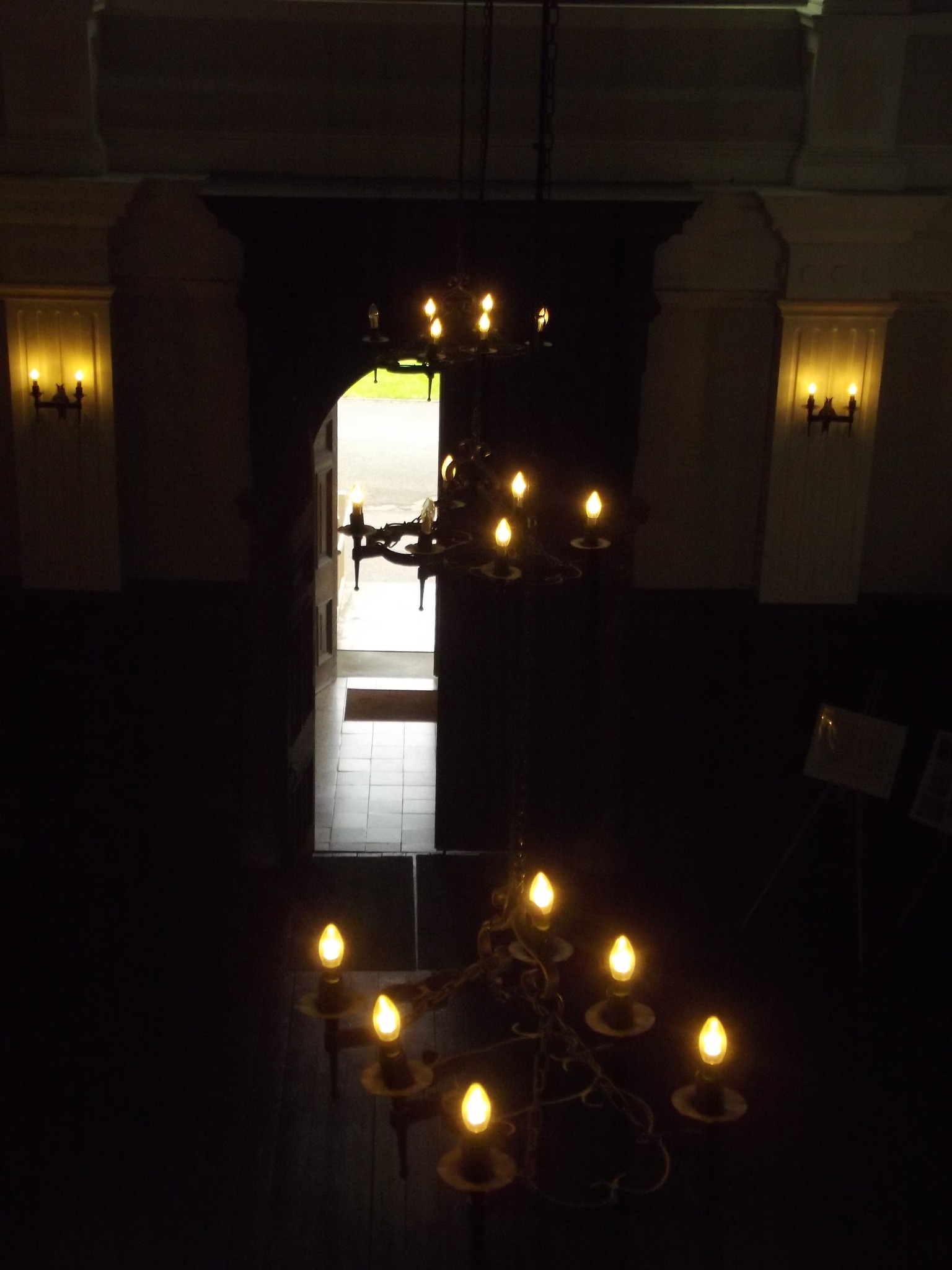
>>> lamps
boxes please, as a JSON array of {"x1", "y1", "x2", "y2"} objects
[
  {"x1": 30, "y1": 365, "x2": 86, "y2": 425},
  {"x1": 343, "y1": 459, "x2": 609, "y2": 604},
  {"x1": 804, "y1": 377, "x2": 859, "y2": 434},
  {"x1": 362, "y1": 287, "x2": 552, "y2": 370},
  {"x1": 306, "y1": 843, "x2": 748, "y2": 1270}
]
